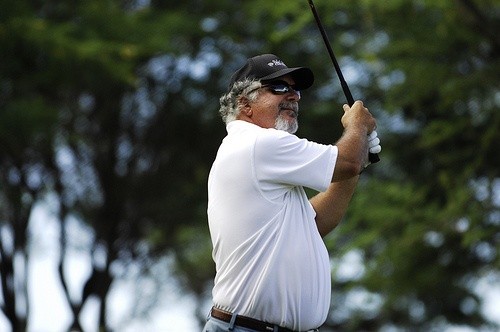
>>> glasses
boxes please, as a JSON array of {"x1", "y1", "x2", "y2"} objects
[{"x1": 245, "y1": 80, "x2": 303, "y2": 96}]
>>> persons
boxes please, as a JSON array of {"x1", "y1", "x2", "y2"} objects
[{"x1": 204, "y1": 54, "x2": 382, "y2": 332}]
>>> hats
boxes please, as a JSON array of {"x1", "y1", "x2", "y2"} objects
[{"x1": 228, "y1": 53, "x2": 315, "y2": 93}]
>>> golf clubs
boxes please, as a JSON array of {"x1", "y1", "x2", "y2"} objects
[{"x1": 307, "y1": 0, "x2": 383, "y2": 164}]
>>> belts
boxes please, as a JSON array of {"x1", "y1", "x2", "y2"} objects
[{"x1": 211, "y1": 306, "x2": 295, "y2": 332}]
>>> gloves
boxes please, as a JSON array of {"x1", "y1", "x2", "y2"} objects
[{"x1": 366, "y1": 132, "x2": 381, "y2": 166}]
